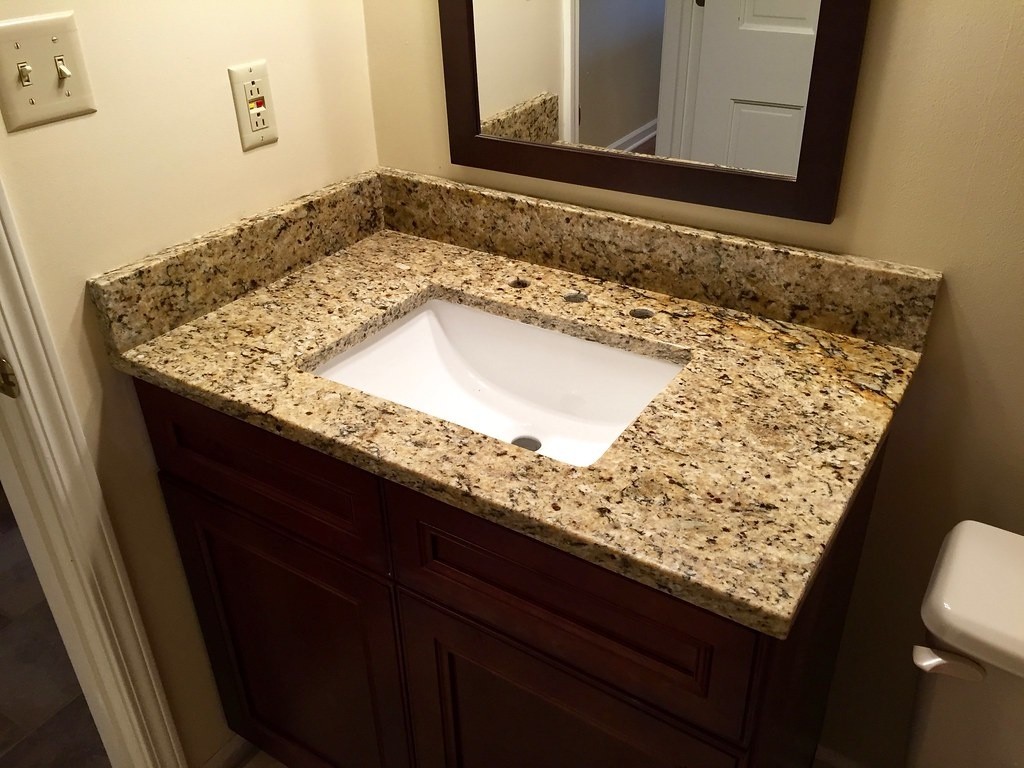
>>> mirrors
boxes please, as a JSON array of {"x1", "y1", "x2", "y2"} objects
[{"x1": 437, "y1": 0, "x2": 870, "y2": 224}]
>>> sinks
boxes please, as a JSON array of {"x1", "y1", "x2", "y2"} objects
[{"x1": 295, "y1": 281, "x2": 695, "y2": 470}]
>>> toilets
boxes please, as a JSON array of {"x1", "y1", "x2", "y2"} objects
[{"x1": 903, "y1": 518, "x2": 1024, "y2": 768}]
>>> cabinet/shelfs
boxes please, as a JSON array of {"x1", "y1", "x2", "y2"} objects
[{"x1": 131, "y1": 375, "x2": 898, "y2": 768}]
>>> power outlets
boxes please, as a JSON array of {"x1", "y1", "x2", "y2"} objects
[{"x1": 228, "y1": 67, "x2": 280, "y2": 151}]
